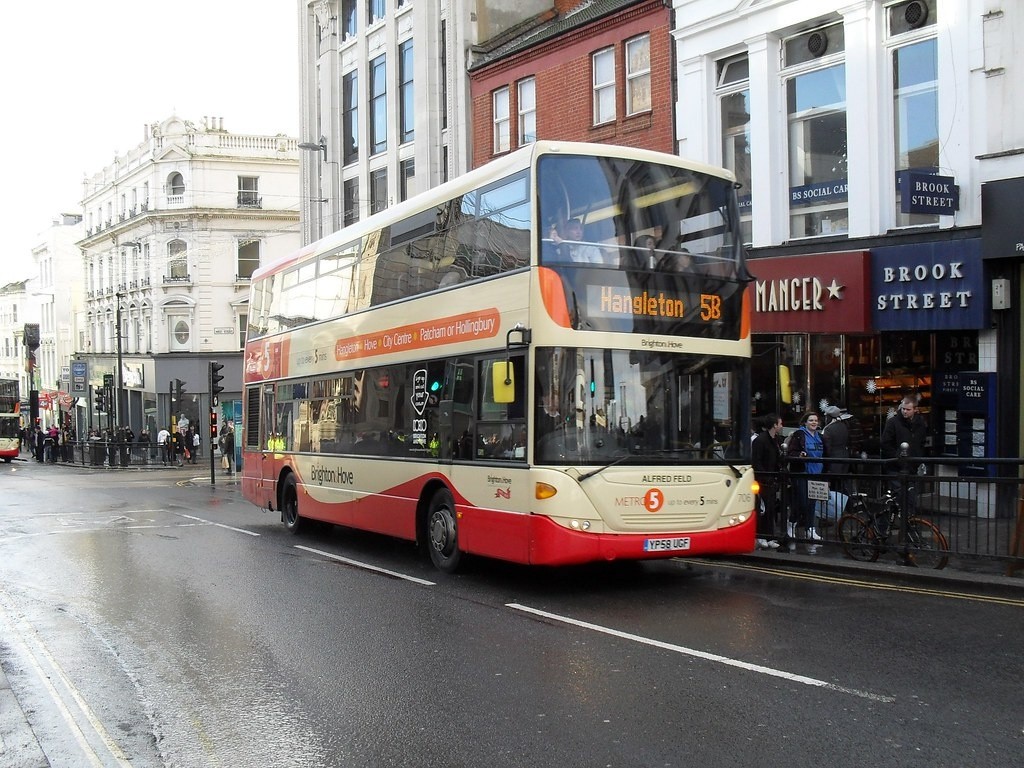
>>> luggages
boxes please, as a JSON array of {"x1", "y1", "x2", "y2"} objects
[{"x1": 60, "y1": 445, "x2": 74, "y2": 464}]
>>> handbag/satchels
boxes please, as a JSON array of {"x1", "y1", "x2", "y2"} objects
[
  {"x1": 222, "y1": 454, "x2": 229, "y2": 470},
  {"x1": 184, "y1": 449, "x2": 191, "y2": 459},
  {"x1": 815, "y1": 491, "x2": 849, "y2": 523}
]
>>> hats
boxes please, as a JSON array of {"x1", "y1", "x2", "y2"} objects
[
  {"x1": 34, "y1": 426, "x2": 41, "y2": 430},
  {"x1": 824, "y1": 406, "x2": 840, "y2": 417}
]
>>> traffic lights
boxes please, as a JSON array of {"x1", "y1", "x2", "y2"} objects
[
  {"x1": 429, "y1": 380, "x2": 441, "y2": 392},
  {"x1": 95, "y1": 388, "x2": 105, "y2": 412},
  {"x1": 30, "y1": 391, "x2": 39, "y2": 417},
  {"x1": 175, "y1": 379, "x2": 187, "y2": 412},
  {"x1": 212, "y1": 362, "x2": 224, "y2": 407},
  {"x1": 211, "y1": 413, "x2": 217, "y2": 424},
  {"x1": 212, "y1": 426, "x2": 217, "y2": 437}
]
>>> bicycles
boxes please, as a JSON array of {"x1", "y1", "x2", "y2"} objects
[{"x1": 838, "y1": 486, "x2": 949, "y2": 570}]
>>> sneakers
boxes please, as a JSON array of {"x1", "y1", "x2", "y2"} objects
[
  {"x1": 804, "y1": 527, "x2": 823, "y2": 540},
  {"x1": 806, "y1": 544, "x2": 823, "y2": 555},
  {"x1": 756, "y1": 539, "x2": 769, "y2": 548},
  {"x1": 873, "y1": 513, "x2": 887, "y2": 537},
  {"x1": 905, "y1": 541, "x2": 933, "y2": 552},
  {"x1": 767, "y1": 540, "x2": 780, "y2": 548},
  {"x1": 787, "y1": 520, "x2": 797, "y2": 538},
  {"x1": 788, "y1": 542, "x2": 796, "y2": 551}
]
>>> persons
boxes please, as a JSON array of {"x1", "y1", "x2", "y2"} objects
[
  {"x1": 157, "y1": 425, "x2": 202, "y2": 466},
  {"x1": 558, "y1": 218, "x2": 604, "y2": 264},
  {"x1": 879, "y1": 395, "x2": 926, "y2": 544},
  {"x1": 219, "y1": 425, "x2": 234, "y2": 475},
  {"x1": 19, "y1": 424, "x2": 76, "y2": 463},
  {"x1": 751, "y1": 405, "x2": 848, "y2": 554},
  {"x1": 625, "y1": 235, "x2": 656, "y2": 270},
  {"x1": 87, "y1": 425, "x2": 153, "y2": 466}
]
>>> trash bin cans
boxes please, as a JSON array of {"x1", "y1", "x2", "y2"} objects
[{"x1": 88, "y1": 437, "x2": 106, "y2": 466}]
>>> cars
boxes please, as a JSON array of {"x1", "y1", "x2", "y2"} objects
[{"x1": 848, "y1": 365, "x2": 904, "y2": 390}]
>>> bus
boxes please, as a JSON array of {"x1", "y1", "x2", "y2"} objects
[
  {"x1": 242, "y1": 140, "x2": 797, "y2": 577},
  {"x1": 0, "y1": 377, "x2": 21, "y2": 463}
]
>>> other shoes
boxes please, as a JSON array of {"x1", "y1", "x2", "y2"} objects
[
  {"x1": 37, "y1": 461, "x2": 44, "y2": 463},
  {"x1": 192, "y1": 462, "x2": 198, "y2": 464},
  {"x1": 188, "y1": 460, "x2": 190, "y2": 463},
  {"x1": 226, "y1": 470, "x2": 232, "y2": 475},
  {"x1": 162, "y1": 461, "x2": 167, "y2": 463}
]
